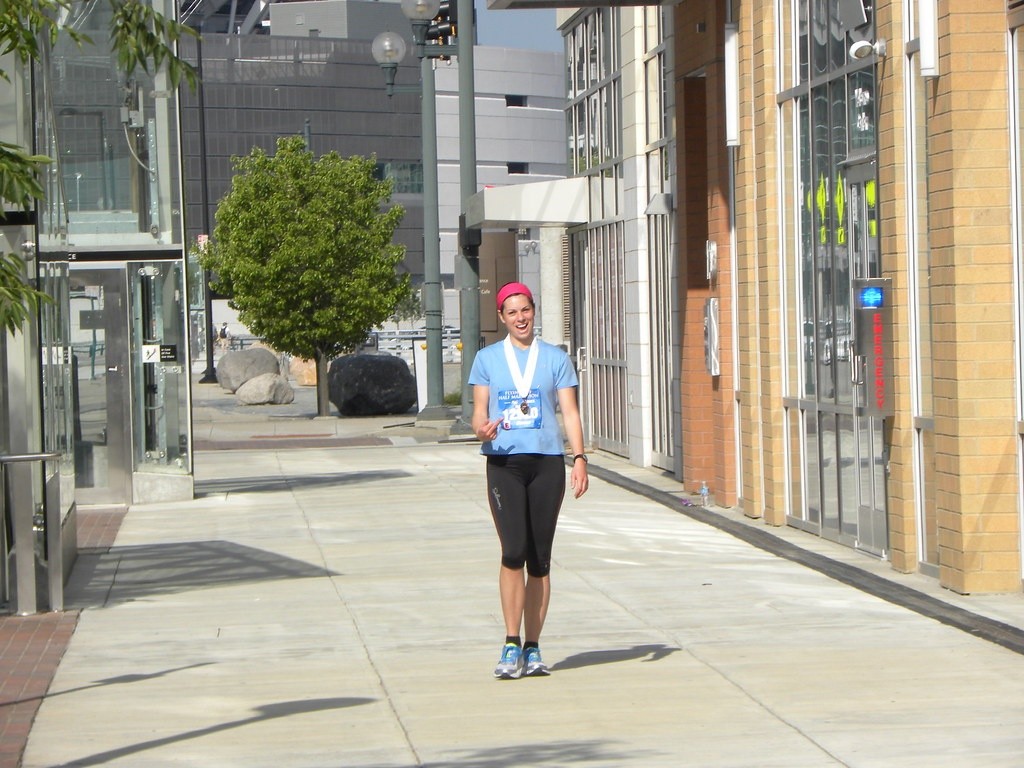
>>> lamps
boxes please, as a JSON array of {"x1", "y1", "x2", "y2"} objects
[{"x1": 849, "y1": 38, "x2": 886, "y2": 59}]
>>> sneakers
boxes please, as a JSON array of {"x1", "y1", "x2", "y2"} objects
[{"x1": 494, "y1": 643, "x2": 551, "y2": 678}]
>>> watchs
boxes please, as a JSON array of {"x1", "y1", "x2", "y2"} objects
[{"x1": 573, "y1": 453, "x2": 587, "y2": 464}]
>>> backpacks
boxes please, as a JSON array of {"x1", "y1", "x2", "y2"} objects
[{"x1": 220, "y1": 327, "x2": 227, "y2": 338}]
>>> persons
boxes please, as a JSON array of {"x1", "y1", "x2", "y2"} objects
[
  {"x1": 468, "y1": 281, "x2": 588, "y2": 679},
  {"x1": 200, "y1": 322, "x2": 232, "y2": 353}
]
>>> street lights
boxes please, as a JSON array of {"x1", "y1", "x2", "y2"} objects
[
  {"x1": 371, "y1": 32, "x2": 456, "y2": 420},
  {"x1": 401, "y1": 0, "x2": 481, "y2": 442},
  {"x1": 58, "y1": 108, "x2": 109, "y2": 211}
]
